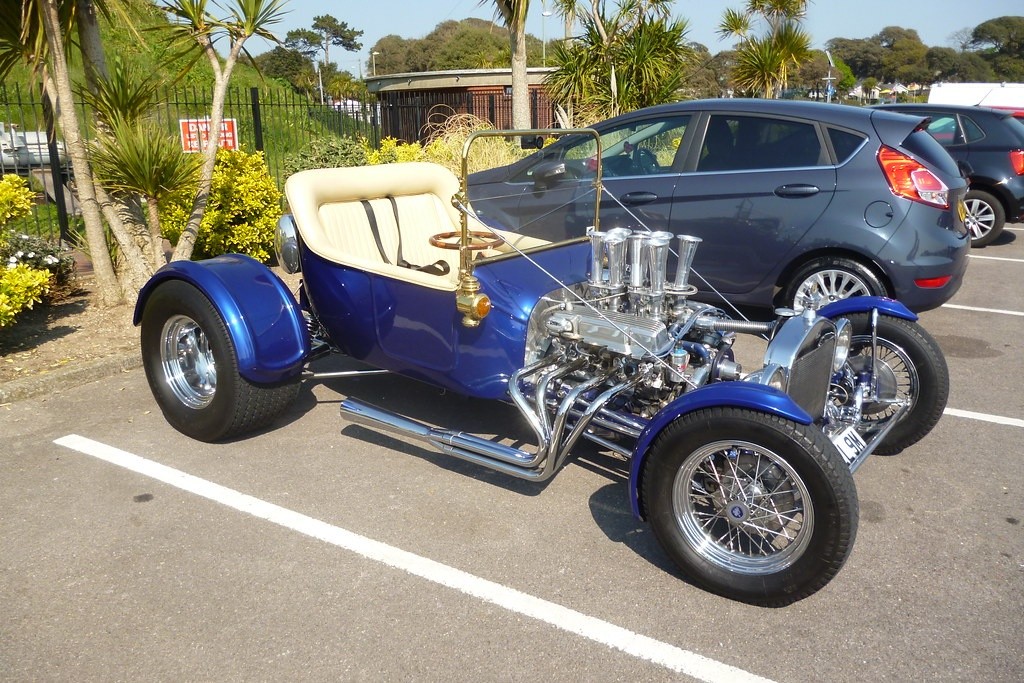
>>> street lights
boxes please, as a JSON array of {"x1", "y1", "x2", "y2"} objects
[
  {"x1": 541, "y1": 10, "x2": 554, "y2": 68},
  {"x1": 372, "y1": 49, "x2": 381, "y2": 78}
]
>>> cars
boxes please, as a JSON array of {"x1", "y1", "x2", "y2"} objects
[
  {"x1": 463, "y1": 98, "x2": 973, "y2": 329},
  {"x1": 755, "y1": 101, "x2": 1023, "y2": 250},
  {"x1": 930, "y1": 106, "x2": 1024, "y2": 140},
  {"x1": 134, "y1": 126, "x2": 952, "y2": 608}
]
